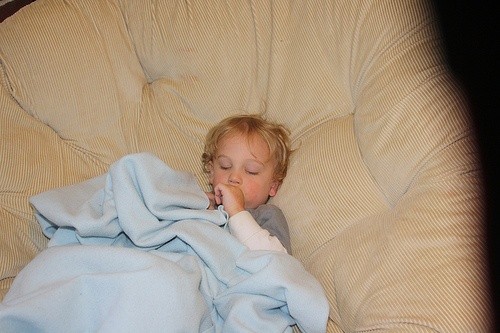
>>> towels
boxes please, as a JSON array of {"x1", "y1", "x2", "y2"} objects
[{"x1": 1, "y1": 153, "x2": 331, "y2": 333}]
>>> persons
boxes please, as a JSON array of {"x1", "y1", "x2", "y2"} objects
[{"x1": 200, "y1": 112, "x2": 293, "y2": 255}]
